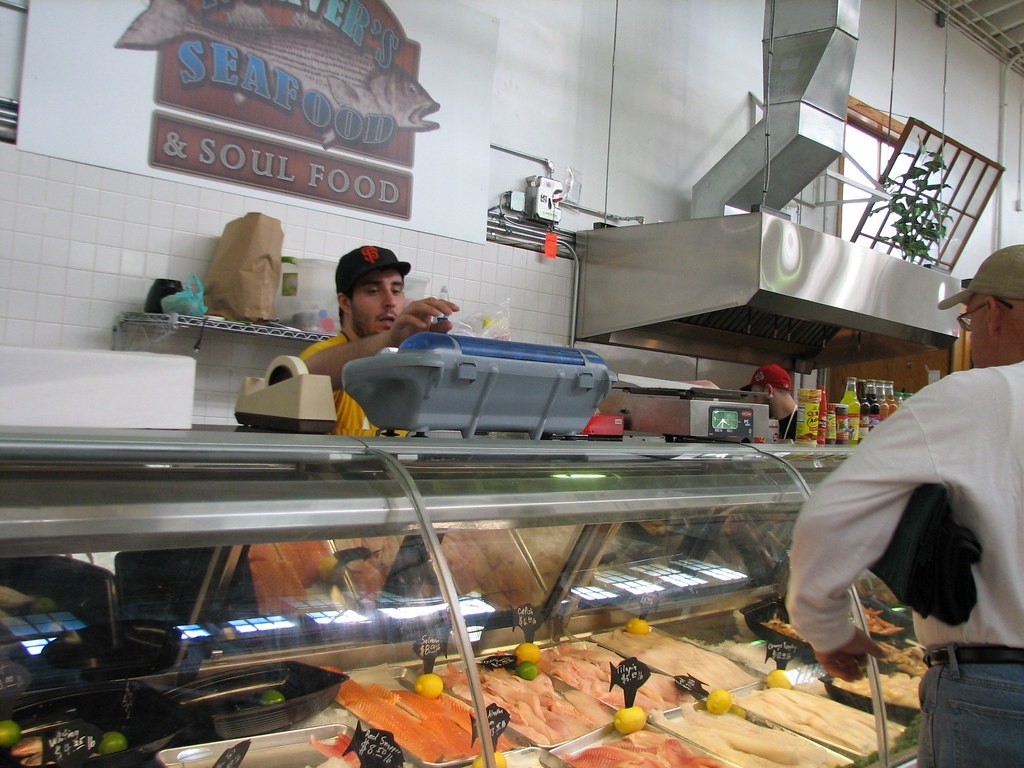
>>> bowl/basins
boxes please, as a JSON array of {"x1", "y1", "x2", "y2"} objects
[
  {"x1": 0, "y1": 545, "x2": 260, "y2": 645},
  {"x1": 0, "y1": 679, "x2": 193, "y2": 768},
  {"x1": 627, "y1": 519, "x2": 687, "y2": 539},
  {"x1": 738, "y1": 598, "x2": 816, "y2": 659},
  {"x1": 860, "y1": 598, "x2": 913, "y2": 639},
  {"x1": 40, "y1": 619, "x2": 182, "y2": 678},
  {"x1": 818, "y1": 643, "x2": 920, "y2": 727},
  {"x1": 162, "y1": 660, "x2": 349, "y2": 740}
]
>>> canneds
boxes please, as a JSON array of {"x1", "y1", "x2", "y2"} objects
[{"x1": 826, "y1": 403, "x2": 849, "y2": 445}]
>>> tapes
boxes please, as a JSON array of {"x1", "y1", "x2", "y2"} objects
[{"x1": 262, "y1": 355, "x2": 309, "y2": 388}]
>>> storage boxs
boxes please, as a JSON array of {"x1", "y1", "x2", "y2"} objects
[
  {"x1": 0, "y1": 346, "x2": 196, "y2": 430},
  {"x1": 277, "y1": 255, "x2": 431, "y2": 335}
]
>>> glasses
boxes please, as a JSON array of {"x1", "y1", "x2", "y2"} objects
[{"x1": 956, "y1": 297, "x2": 1014, "y2": 331}]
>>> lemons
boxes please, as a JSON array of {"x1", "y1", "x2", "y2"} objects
[
  {"x1": 259, "y1": 690, "x2": 285, "y2": 706},
  {"x1": 764, "y1": 670, "x2": 793, "y2": 689},
  {"x1": 706, "y1": 689, "x2": 733, "y2": 715},
  {"x1": 316, "y1": 556, "x2": 339, "y2": 581},
  {"x1": 614, "y1": 706, "x2": 646, "y2": 734},
  {"x1": 514, "y1": 642, "x2": 540, "y2": 663},
  {"x1": 95, "y1": 731, "x2": 128, "y2": 754},
  {"x1": 625, "y1": 618, "x2": 648, "y2": 635},
  {"x1": 471, "y1": 751, "x2": 508, "y2": 768},
  {"x1": 414, "y1": 673, "x2": 444, "y2": 698},
  {"x1": 515, "y1": 660, "x2": 538, "y2": 680},
  {"x1": 0, "y1": 720, "x2": 22, "y2": 748}
]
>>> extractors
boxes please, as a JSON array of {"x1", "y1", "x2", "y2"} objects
[{"x1": 575, "y1": 0, "x2": 960, "y2": 367}]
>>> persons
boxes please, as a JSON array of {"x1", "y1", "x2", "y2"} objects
[
  {"x1": 740, "y1": 363, "x2": 799, "y2": 445},
  {"x1": 787, "y1": 244, "x2": 1024, "y2": 768},
  {"x1": 298, "y1": 246, "x2": 460, "y2": 437}
]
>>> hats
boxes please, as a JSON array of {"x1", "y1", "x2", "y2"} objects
[
  {"x1": 335, "y1": 246, "x2": 411, "y2": 296},
  {"x1": 740, "y1": 364, "x2": 793, "y2": 397},
  {"x1": 937, "y1": 243, "x2": 1024, "y2": 310}
]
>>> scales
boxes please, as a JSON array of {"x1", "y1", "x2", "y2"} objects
[{"x1": 610, "y1": 384, "x2": 772, "y2": 443}]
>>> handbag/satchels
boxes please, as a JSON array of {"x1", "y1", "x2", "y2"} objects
[
  {"x1": 869, "y1": 483, "x2": 982, "y2": 626},
  {"x1": 203, "y1": 212, "x2": 284, "y2": 322},
  {"x1": 160, "y1": 274, "x2": 208, "y2": 317}
]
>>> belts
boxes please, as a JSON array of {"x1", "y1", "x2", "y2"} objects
[{"x1": 927, "y1": 646, "x2": 1023, "y2": 666}]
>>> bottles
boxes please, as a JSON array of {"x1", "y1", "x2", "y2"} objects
[
  {"x1": 866, "y1": 379, "x2": 881, "y2": 431},
  {"x1": 904, "y1": 393, "x2": 913, "y2": 400},
  {"x1": 274, "y1": 257, "x2": 299, "y2": 321},
  {"x1": 817, "y1": 385, "x2": 827, "y2": 445},
  {"x1": 876, "y1": 380, "x2": 889, "y2": 421},
  {"x1": 840, "y1": 377, "x2": 860, "y2": 444},
  {"x1": 795, "y1": 389, "x2": 821, "y2": 444},
  {"x1": 856, "y1": 379, "x2": 870, "y2": 443},
  {"x1": 895, "y1": 392, "x2": 903, "y2": 407},
  {"x1": 432, "y1": 286, "x2": 448, "y2": 324},
  {"x1": 885, "y1": 381, "x2": 895, "y2": 416}
]
